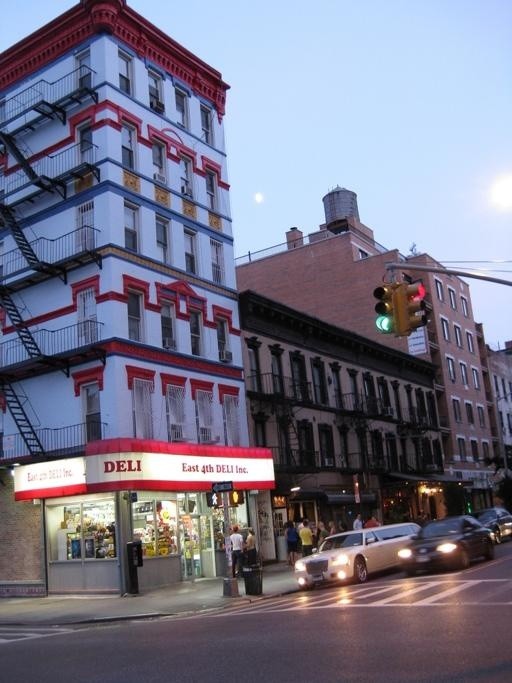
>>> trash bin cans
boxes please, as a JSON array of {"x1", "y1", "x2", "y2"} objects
[{"x1": 242, "y1": 563, "x2": 264, "y2": 597}]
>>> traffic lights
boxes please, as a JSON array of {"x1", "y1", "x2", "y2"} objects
[
  {"x1": 206, "y1": 492, "x2": 222, "y2": 507},
  {"x1": 373, "y1": 286, "x2": 395, "y2": 334},
  {"x1": 229, "y1": 491, "x2": 245, "y2": 504},
  {"x1": 398, "y1": 282, "x2": 426, "y2": 334}
]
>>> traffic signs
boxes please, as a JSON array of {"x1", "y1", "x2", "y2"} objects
[{"x1": 212, "y1": 481, "x2": 232, "y2": 493}]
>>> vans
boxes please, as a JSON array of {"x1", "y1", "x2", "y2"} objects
[{"x1": 294, "y1": 522, "x2": 423, "y2": 590}]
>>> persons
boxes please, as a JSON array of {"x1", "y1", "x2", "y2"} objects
[
  {"x1": 184, "y1": 535, "x2": 195, "y2": 576},
  {"x1": 229, "y1": 526, "x2": 244, "y2": 578},
  {"x1": 353, "y1": 512, "x2": 363, "y2": 530},
  {"x1": 282, "y1": 516, "x2": 346, "y2": 565},
  {"x1": 244, "y1": 527, "x2": 256, "y2": 564},
  {"x1": 363, "y1": 513, "x2": 381, "y2": 527}
]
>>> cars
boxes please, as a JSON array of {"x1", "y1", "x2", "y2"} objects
[
  {"x1": 398, "y1": 514, "x2": 495, "y2": 577},
  {"x1": 474, "y1": 507, "x2": 511, "y2": 543}
]
